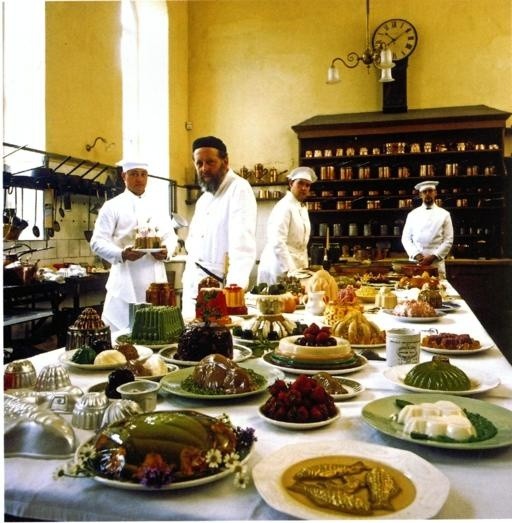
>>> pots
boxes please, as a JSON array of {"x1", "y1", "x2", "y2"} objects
[
  {"x1": 4, "y1": 143, "x2": 108, "y2": 189},
  {"x1": 303, "y1": 187, "x2": 491, "y2": 211},
  {"x1": 21, "y1": 256, "x2": 42, "y2": 284},
  {"x1": 318, "y1": 164, "x2": 496, "y2": 180}
]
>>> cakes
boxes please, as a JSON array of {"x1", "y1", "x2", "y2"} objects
[{"x1": 271, "y1": 323, "x2": 358, "y2": 369}]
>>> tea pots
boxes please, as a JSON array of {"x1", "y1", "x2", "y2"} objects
[{"x1": 236, "y1": 163, "x2": 291, "y2": 183}]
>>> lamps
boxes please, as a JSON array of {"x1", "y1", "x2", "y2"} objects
[
  {"x1": 84, "y1": 135, "x2": 117, "y2": 154},
  {"x1": 323, "y1": 0, "x2": 397, "y2": 86}
]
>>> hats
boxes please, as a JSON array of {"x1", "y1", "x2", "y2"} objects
[
  {"x1": 288, "y1": 166, "x2": 317, "y2": 183},
  {"x1": 414, "y1": 181, "x2": 438, "y2": 191},
  {"x1": 116, "y1": 157, "x2": 150, "y2": 174}
]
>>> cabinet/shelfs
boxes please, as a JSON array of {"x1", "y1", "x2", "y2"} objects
[
  {"x1": 291, "y1": 103, "x2": 512, "y2": 257},
  {"x1": 185, "y1": 180, "x2": 289, "y2": 203}
]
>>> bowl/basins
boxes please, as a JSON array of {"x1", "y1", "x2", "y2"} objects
[
  {"x1": 69, "y1": 395, "x2": 105, "y2": 429},
  {"x1": 96, "y1": 399, "x2": 140, "y2": 422},
  {"x1": 48, "y1": 385, "x2": 83, "y2": 418},
  {"x1": 5, "y1": 359, "x2": 36, "y2": 389},
  {"x1": 35, "y1": 362, "x2": 71, "y2": 390}
]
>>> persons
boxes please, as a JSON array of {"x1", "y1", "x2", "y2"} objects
[
  {"x1": 400, "y1": 181, "x2": 454, "y2": 279},
  {"x1": 257, "y1": 166, "x2": 318, "y2": 287},
  {"x1": 180, "y1": 135, "x2": 258, "y2": 329},
  {"x1": 88, "y1": 152, "x2": 179, "y2": 333}
]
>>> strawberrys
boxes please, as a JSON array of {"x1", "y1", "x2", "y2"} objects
[{"x1": 262, "y1": 375, "x2": 336, "y2": 423}]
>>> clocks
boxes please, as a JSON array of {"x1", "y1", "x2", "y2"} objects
[{"x1": 372, "y1": 16, "x2": 419, "y2": 62}]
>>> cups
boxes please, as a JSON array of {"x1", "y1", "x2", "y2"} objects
[
  {"x1": 257, "y1": 189, "x2": 282, "y2": 200},
  {"x1": 317, "y1": 221, "x2": 490, "y2": 234},
  {"x1": 113, "y1": 374, "x2": 160, "y2": 415},
  {"x1": 382, "y1": 325, "x2": 422, "y2": 368}
]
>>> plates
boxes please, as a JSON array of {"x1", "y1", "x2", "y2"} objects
[
  {"x1": 252, "y1": 437, "x2": 448, "y2": 518},
  {"x1": 306, "y1": 371, "x2": 362, "y2": 400},
  {"x1": 414, "y1": 326, "x2": 494, "y2": 354},
  {"x1": 128, "y1": 269, "x2": 476, "y2": 328},
  {"x1": 156, "y1": 341, "x2": 254, "y2": 366},
  {"x1": 59, "y1": 346, "x2": 151, "y2": 370},
  {"x1": 110, "y1": 363, "x2": 176, "y2": 382},
  {"x1": 160, "y1": 365, "x2": 276, "y2": 401},
  {"x1": 341, "y1": 329, "x2": 385, "y2": 348},
  {"x1": 75, "y1": 425, "x2": 260, "y2": 491},
  {"x1": 260, "y1": 351, "x2": 370, "y2": 374},
  {"x1": 256, "y1": 400, "x2": 341, "y2": 432},
  {"x1": 118, "y1": 332, "x2": 180, "y2": 347},
  {"x1": 228, "y1": 325, "x2": 285, "y2": 344},
  {"x1": 360, "y1": 391, "x2": 512, "y2": 451},
  {"x1": 380, "y1": 360, "x2": 504, "y2": 398}
]
